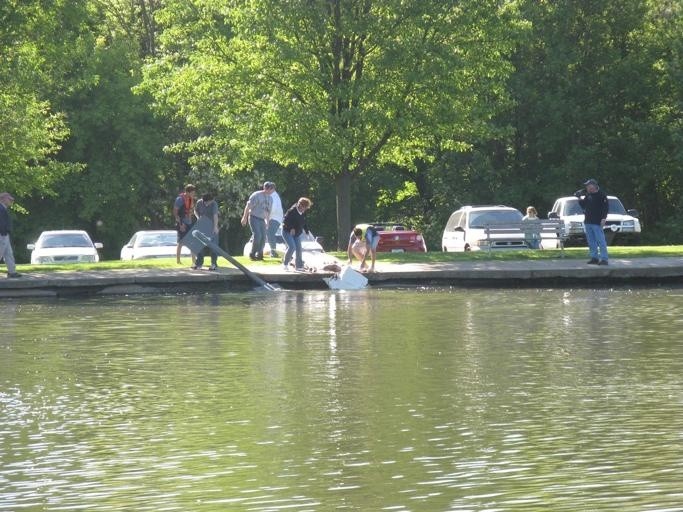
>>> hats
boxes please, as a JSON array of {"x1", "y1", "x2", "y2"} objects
[
  {"x1": 0, "y1": 192, "x2": 14, "y2": 200},
  {"x1": 584, "y1": 179, "x2": 597, "y2": 186}
]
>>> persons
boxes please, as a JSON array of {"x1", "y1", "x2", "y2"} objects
[
  {"x1": 518, "y1": 206, "x2": 542, "y2": 250},
  {"x1": 575, "y1": 178, "x2": 609, "y2": 266},
  {"x1": 0, "y1": 192, "x2": 22, "y2": 278},
  {"x1": 189, "y1": 193, "x2": 218, "y2": 270},
  {"x1": 280, "y1": 196, "x2": 312, "y2": 272},
  {"x1": 266, "y1": 190, "x2": 283, "y2": 258},
  {"x1": 171, "y1": 183, "x2": 196, "y2": 264},
  {"x1": 346, "y1": 223, "x2": 380, "y2": 272},
  {"x1": 239, "y1": 181, "x2": 276, "y2": 261}
]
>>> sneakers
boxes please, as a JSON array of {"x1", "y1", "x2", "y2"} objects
[
  {"x1": 209, "y1": 264, "x2": 217, "y2": 270},
  {"x1": 191, "y1": 264, "x2": 202, "y2": 270},
  {"x1": 8, "y1": 272, "x2": 21, "y2": 277},
  {"x1": 271, "y1": 250, "x2": 278, "y2": 257},
  {"x1": 250, "y1": 255, "x2": 262, "y2": 260},
  {"x1": 588, "y1": 258, "x2": 608, "y2": 265}
]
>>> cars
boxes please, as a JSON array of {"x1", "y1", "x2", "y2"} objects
[
  {"x1": 241, "y1": 227, "x2": 325, "y2": 257},
  {"x1": 23, "y1": 230, "x2": 105, "y2": 267},
  {"x1": 120, "y1": 228, "x2": 199, "y2": 263}
]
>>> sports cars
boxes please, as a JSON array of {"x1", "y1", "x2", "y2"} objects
[{"x1": 350, "y1": 221, "x2": 432, "y2": 255}]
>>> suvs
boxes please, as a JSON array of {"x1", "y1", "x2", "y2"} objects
[
  {"x1": 547, "y1": 195, "x2": 643, "y2": 247},
  {"x1": 440, "y1": 204, "x2": 542, "y2": 252}
]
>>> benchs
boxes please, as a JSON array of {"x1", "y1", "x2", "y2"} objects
[{"x1": 483, "y1": 220, "x2": 569, "y2": 260}]
